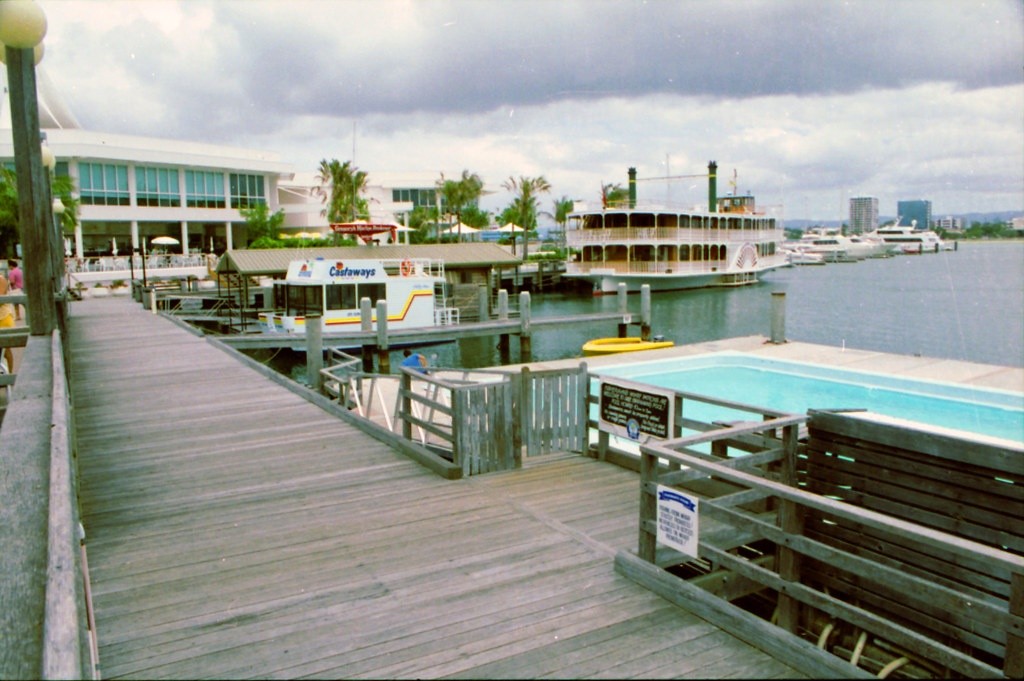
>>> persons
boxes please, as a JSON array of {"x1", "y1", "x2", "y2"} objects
[
  {"x1": 401, "y1": 348, "x2": 430, "y2": 376},
  {"x1": 0, "y1": 274, "x2": 15, "y2": 374},
  {"x1": 8, "y1": 259, "x2": 28, "y2": 321}
]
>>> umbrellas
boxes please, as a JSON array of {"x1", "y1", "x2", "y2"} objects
[
  {"x1": 112, "y1": 235, "x2": 120, "y2": 256},
  {"x1": 64, "y1": 237, "x2": 73, "y2": 258},
  {"x1": 150, "y1": 235, "x2": 180, "y2": 255},
  {"x1": 210, "y1": 236, "x2": 214, "y2": 253}
]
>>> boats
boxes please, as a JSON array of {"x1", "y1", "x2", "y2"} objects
[{"x1": 255, "y1": 162, "x2": 953, "y2": 460}]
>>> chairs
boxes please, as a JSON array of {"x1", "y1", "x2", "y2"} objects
[{"x1": 65, "y1": 253, "x2": 217, "y2": 274}]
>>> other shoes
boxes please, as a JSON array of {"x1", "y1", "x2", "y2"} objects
[{"x1": 14, "y1": 317, "x2": 21, "y2": 321}]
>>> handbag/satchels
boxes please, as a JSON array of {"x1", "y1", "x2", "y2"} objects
[{"x1": 8, "y1": 280, "x2": 23, "y2": 295}]
